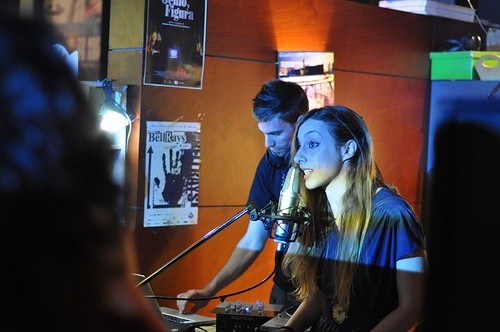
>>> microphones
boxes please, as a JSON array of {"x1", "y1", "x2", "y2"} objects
[{"x1": 273, "y1": 167, "x2": 302, "y2": 260}]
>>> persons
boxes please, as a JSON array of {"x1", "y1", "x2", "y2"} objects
[
  {"x1": 173, "y1": 81, "x2": 312, "y2": 317},
  {"x1": 266, "y1": 104, "x2": 431, "y2": 332},
  {"x1": 4, "y1": 3, "x2": 172, "y2": 331}
]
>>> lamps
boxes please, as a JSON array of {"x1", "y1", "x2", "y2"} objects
[{"x1": 98, "y1": 78, "x2": 133, "y2": 131}]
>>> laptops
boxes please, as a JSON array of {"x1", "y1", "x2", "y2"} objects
[{"x1": 131, "y1": 272, "x2": 216, "y2": 328}]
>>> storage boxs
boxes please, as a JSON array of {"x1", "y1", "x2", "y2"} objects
[{"x1": 428, "y1": 50, "x2": 479, "y2": 80}]
